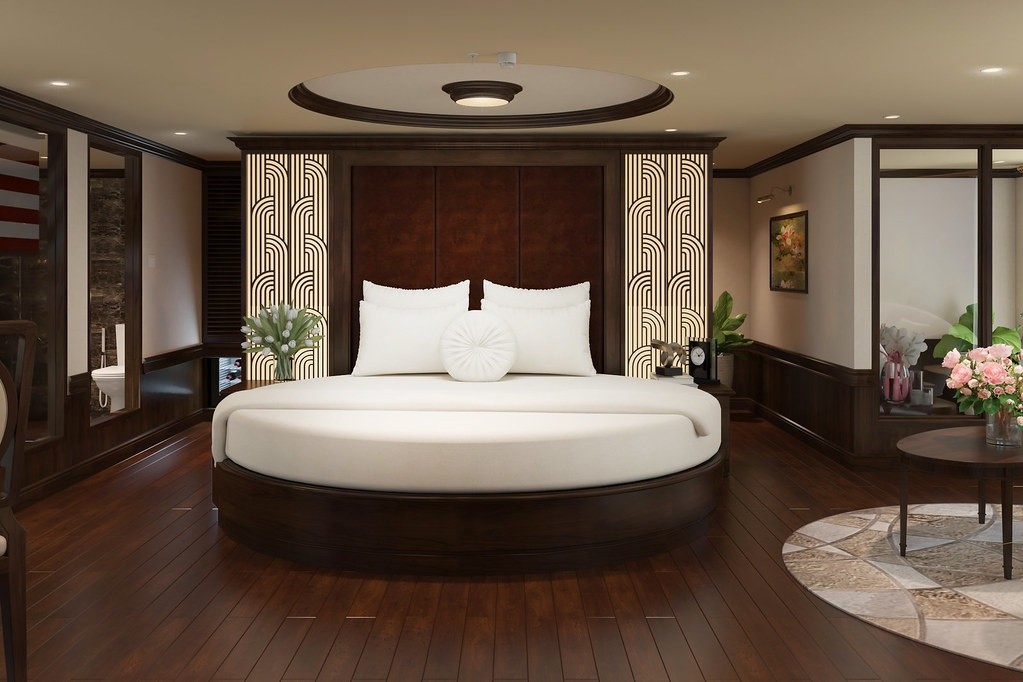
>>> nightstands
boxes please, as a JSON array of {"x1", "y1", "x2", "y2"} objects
[
  {"x1": 219, "y1": 380, "x2": 280, "y2": 400},
  {"x1": 696, "y1": 382, "x2": 736, "y2": 478}
]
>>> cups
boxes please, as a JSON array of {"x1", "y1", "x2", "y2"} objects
[{"x1": 909, "y1": 370, "x2": 934, "y2": 407}]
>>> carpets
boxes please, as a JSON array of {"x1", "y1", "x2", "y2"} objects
[{"x1": 782, "y1": 503, "x2": 1023, "y2": 673}]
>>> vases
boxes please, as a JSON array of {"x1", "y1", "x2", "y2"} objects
[
  {"x1": 985, "y1": 405, "x2": 1023, "y2": 448},
  {"x1": 273, "y1": 354, "x2": 294, "y2": 382},
  {"x1": 882, "y1": 361, "x2": 910, "y2": 405}
]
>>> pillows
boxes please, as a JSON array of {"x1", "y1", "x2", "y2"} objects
[
  {"x1": 482, "y1": 279, "x2": 590, "y2": 310},
  {"x1": 362, "y1": 280, "x2": 470, "y2": 304},
  {"x1": 352, "y1": 299, "x2": 469, "y2": 376},
  {"x1": 440, "y1": 310, "x2": 517, "y2": 381},
  {"x1": 481, "y1": 299, "x2": 597, "y2": 378}
]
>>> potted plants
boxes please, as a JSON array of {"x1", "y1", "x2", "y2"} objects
[{"x1": 712, "y1": 290, "x2": 754, "y2": 389}]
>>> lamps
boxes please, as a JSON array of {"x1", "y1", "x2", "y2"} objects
[
  {"x1": 757, "y1": 185, "x2": 793, "y2": 204},
  {"x1": 442, "y1": 80, "x2": 523, "y2": 107}
]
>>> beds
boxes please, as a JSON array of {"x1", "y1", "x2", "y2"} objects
[{"x1": 209, "y1": 374, "x2": 728, "y2": 575}]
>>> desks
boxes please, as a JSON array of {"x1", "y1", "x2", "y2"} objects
[
  {"x1": 924, "y1": 365, "x2": 952, "y2": 376},
  {"x1": 879, "y1": 395, "x2": 956, "y2": 416},
  {"x1": 896, "y1": 426, "x2": 1023, "y2": 581}
]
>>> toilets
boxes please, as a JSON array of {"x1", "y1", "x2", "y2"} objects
[{"x1": 90, "y1": 322, "x2": 126, "y2": 414}]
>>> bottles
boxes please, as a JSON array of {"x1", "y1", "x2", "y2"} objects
[{"x1": 226, "y1": 359, "x2": 242, "y2": 380}]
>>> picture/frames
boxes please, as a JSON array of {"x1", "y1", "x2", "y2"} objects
[{"x1": 769, "y1": 210, "x2": 808, "y2": 294}]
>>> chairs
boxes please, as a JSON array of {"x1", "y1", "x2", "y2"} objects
[{"x1": 0, "y1": 321, "x2": 37, "y2": 682}]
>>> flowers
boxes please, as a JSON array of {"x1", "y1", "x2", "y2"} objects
[
  {"x1": 240, "y1": 300, "x2": 325, "y2": 379},
  {"x1": 943, "y1": 344, "x2": 1023, "y2": 426},
  {"x1": 880, "y1": 322, "x2": 927, "y2": 368}
]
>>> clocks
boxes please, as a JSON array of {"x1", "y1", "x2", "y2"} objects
[{"x1": 688, "y1": 336, "x2": 721, "y2": 385}]
